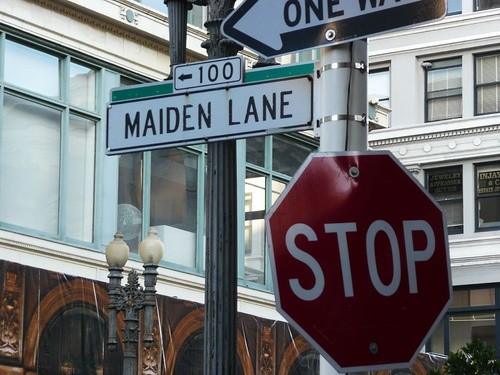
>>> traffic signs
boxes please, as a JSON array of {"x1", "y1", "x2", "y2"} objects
[
  {"x1": 220, "y1": 0, "x2": 448, "y2": 59},
  {"x1": 103, "y1": 53, "x2": 317, "y2": 157}
]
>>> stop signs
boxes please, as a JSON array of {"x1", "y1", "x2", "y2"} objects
[{"x1": 262, "y1": 150, "x2": 455, "y2": 373}]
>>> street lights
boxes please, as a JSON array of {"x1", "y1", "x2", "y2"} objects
[{"x1": 104, "y1": 227, "x2": 164, "y2": 374}]
